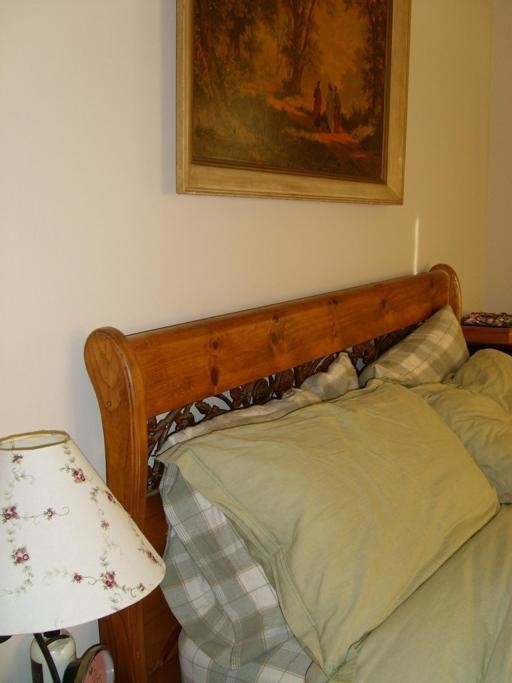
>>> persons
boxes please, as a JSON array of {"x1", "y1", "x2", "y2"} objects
[
  {"x1": 311, "y1": 80, "x2": 323, "y2": 128},
  {"x1": 325, "y1": 82, "x2": 335, "y2": 132},
  {"x1": 333, "y1": 84, "x2": 341, "y2": 130}
]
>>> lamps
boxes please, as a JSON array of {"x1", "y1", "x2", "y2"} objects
[{"x1": 2, "y1": 430, "x2": 165, "y2": 682}]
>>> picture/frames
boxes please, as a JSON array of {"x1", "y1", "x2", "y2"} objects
[{"x1": 172, "y1": 2, "x2": 413, "y2": 207}]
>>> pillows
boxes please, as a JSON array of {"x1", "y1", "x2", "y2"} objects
[{"x1": 156, "y1": 308, "x2": 499, "y2": 680}]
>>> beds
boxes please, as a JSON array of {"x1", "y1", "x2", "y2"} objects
[{"x1": 79, "y1": 264, "x2": 511, "y2": 682}]
